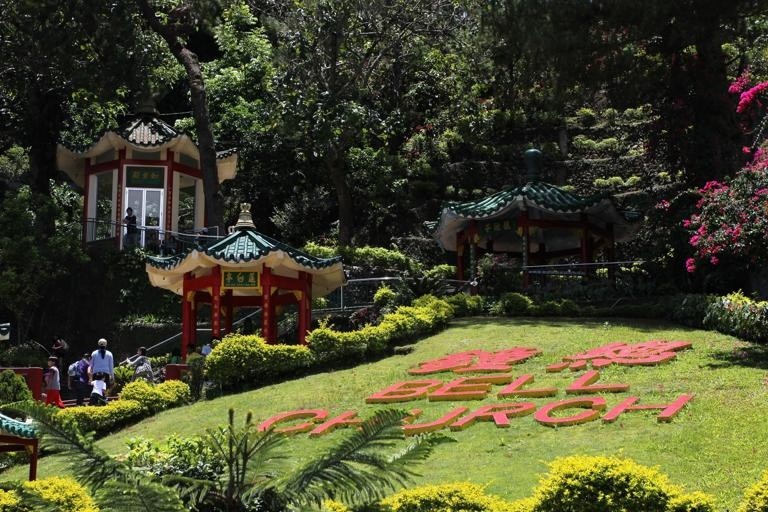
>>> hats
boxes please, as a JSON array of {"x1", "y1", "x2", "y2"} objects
[{"x1": 97, "y1": 338, "x2": 108, "y2": 347}]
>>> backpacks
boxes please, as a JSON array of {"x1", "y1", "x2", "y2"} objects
[{"x1": 68, "y1": 360, "x2": 82, "y2": 377}]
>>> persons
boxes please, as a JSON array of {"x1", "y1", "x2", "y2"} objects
[
  {"x1": 121, "y1": 207, "x2": 210, "y2": 255},
  {"x1": 42, "y1": 335, "x2": 220, "y2": 409}
]
